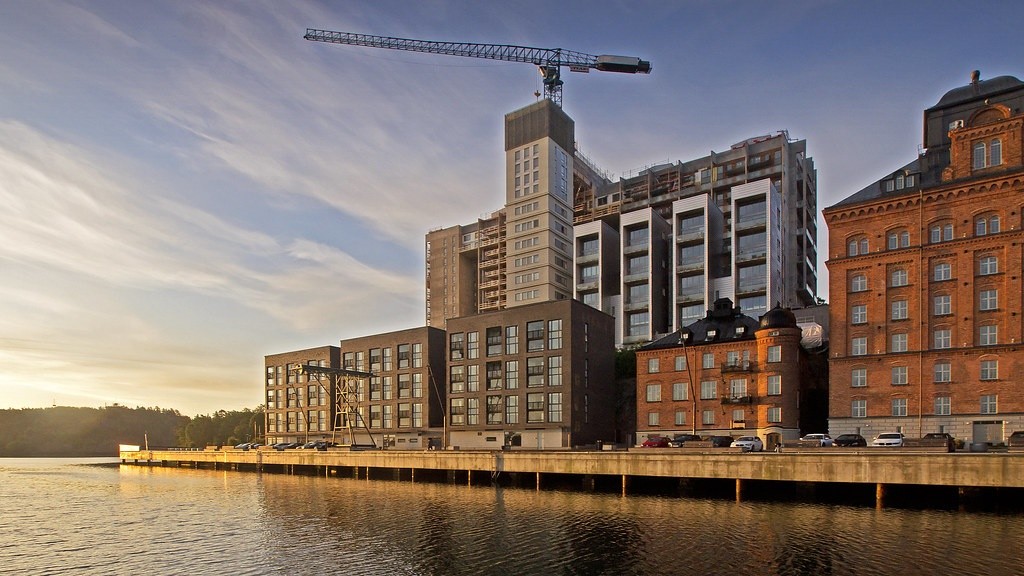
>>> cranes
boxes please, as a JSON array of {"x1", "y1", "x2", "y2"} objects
[{"x1": 303, "y1": 26, "x2": 652, "y2": 109}]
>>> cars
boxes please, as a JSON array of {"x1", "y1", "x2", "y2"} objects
[
  {"x1": 832, "y1": 434, "x2": 867, "y2": 447},
  {"x1": 798, "y1": 433, "x2": 834, "y2": 447},
  {"x1": 922, "y1": 433, "x2": 956, "y2": 453},
  {"x1": 730, "y1": 436, "x2": 764, "y2": 452},
  {"x1": 235, "y1": 440, "x2": 337, "y2": 450},
  {"x1": 668, "y1": 434, "x2": 703, "y2": 448},
  {"x1": 704, "y1": 435, "x2": 733, "y2": 447},
  {"x1": 641, "y1": 437, "x2": 672, "y2": 449},
  {"x1": 871, "y1": 432, "x2": 905, "y2": 447},
  {"x1": 1006, "y1": 431, "x2": 1024, "y2": 449}
]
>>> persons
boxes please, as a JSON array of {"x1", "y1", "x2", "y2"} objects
[
  {"x1": 774, "y1": 441, "x2": 781, "y2": 453},
  {"x1": 427, "y1": 439, "x2": 433, "y2": 451}
]
